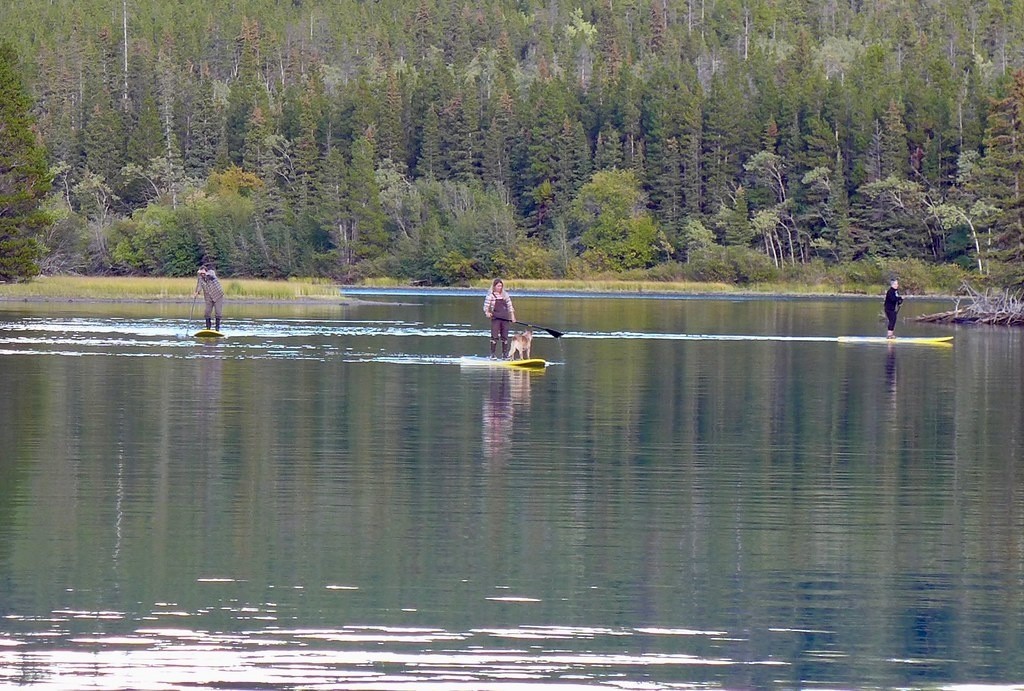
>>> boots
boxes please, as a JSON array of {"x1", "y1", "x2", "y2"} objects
[
  {"x1": 205, "y1": 318, "x2": 211, "y2": 329},
  {"x1": 887, "y1": 330, "x2": 896, "y2": 339},
  {"x1": 216, "y1": 318, "x2": 220, "y2": 331}
]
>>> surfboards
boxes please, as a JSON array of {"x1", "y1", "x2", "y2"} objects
[
  {"x1": 462, "y1": 353, "x2": 547, "y2": 369},
  {"x1": 837, "y1": 334, "x2": 955, "y2": 342},
  {"x1": 192, "y1": 329, "x2": 225, "y2": 336}
]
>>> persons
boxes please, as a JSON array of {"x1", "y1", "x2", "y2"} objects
[
  {"x1": 192, "y1": 265, "x2": 224, "y2": 331},
  {"x1": 483, "y1": 279, "x2": 517, "y2": 361},
  {"x1": 884, "y1": 278, "x2": 904, "y2": 339}
]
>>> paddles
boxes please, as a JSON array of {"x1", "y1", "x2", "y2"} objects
[
  {"x1": 493, "y1": 315, "x2": 564, "y2": 338},
  {"x1": 185, "y1": 274, "x2": 200, "y2": 336}
]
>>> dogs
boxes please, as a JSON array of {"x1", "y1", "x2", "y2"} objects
[{"x1": 508, "y1": 327, "x2": 532, "y2": 361}]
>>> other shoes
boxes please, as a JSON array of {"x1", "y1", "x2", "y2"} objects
[
  {"x1": 490, "y1": 355, "x2": 497, "y2": 359},
  {"x1": 502, "y1": 354, "x2": 510, "y2": 359}
]
